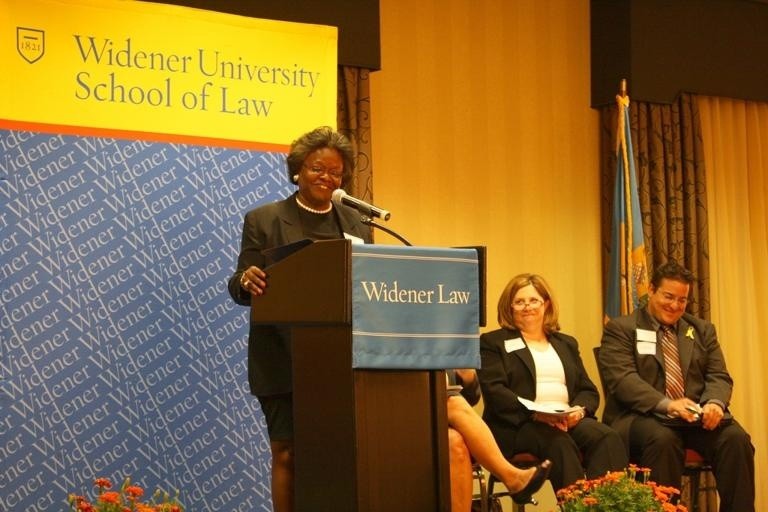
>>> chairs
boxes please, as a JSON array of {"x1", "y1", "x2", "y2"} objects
[
  {"x1": 487, "y1": 451, "x2": 584, "y2": 512},
  {"x1": 597, "y1": 343, "x2": 719, "y2": 512}
]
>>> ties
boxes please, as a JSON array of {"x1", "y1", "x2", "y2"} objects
[{"x1": 660, "y1": 325, "x2": 685, "y2": 419}]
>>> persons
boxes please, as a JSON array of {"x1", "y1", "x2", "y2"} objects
[
  {"x1": 477, "y1": 270, "x2": 627, "y2": 496},
  {"x1": 227, "y1": 125, "x2": 375, "y2": 512},
  {"x1": 598, "y1": 261, "x2": 760, "y2": 512},
  {"x1": 445, "y1": 349, "x2": 553, "y2": 512}
]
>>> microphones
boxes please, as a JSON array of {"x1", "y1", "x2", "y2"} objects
[{"x1": 331, "y1": 189, "x2": 391, "y2": 223}]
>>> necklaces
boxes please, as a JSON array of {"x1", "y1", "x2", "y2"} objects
[{"x1": 296, "y1": 195, "x2": 333, "y2": 213}]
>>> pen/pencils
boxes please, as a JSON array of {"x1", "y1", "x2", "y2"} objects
[
  {"x1": 685, "y1": 407, "x2": 702, "y2": 416},
  {"x1": 555, "y1": 409, "x2": 565, "y2": 412}
]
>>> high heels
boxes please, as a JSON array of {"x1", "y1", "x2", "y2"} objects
[{"x1": 511, "y1": 459, "x2": 552, "y2": 506}]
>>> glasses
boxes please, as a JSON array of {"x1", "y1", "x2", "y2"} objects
[{"x1": 510, "y1": 300, "x2": 543, "y2": 312}]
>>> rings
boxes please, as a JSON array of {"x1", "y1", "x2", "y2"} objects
[{"x1": 244, "y1": 280, "x2": 250, "y2": 288}]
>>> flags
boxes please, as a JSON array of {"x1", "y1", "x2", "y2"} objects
[{"x1": 603, "y1": 97, "x2": 650, "y2": 325}]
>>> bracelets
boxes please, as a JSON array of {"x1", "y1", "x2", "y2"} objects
[{"x1": 577, "y1": 407, "x2": 585, "y2": 418}]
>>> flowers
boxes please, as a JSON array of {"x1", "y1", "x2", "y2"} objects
[
  {"x1": 556, "y1": 463, "x2": 688, "y2": 512},
  {"x1": 67, "y1": 473, "x2": 183, "y2": 512}
]
